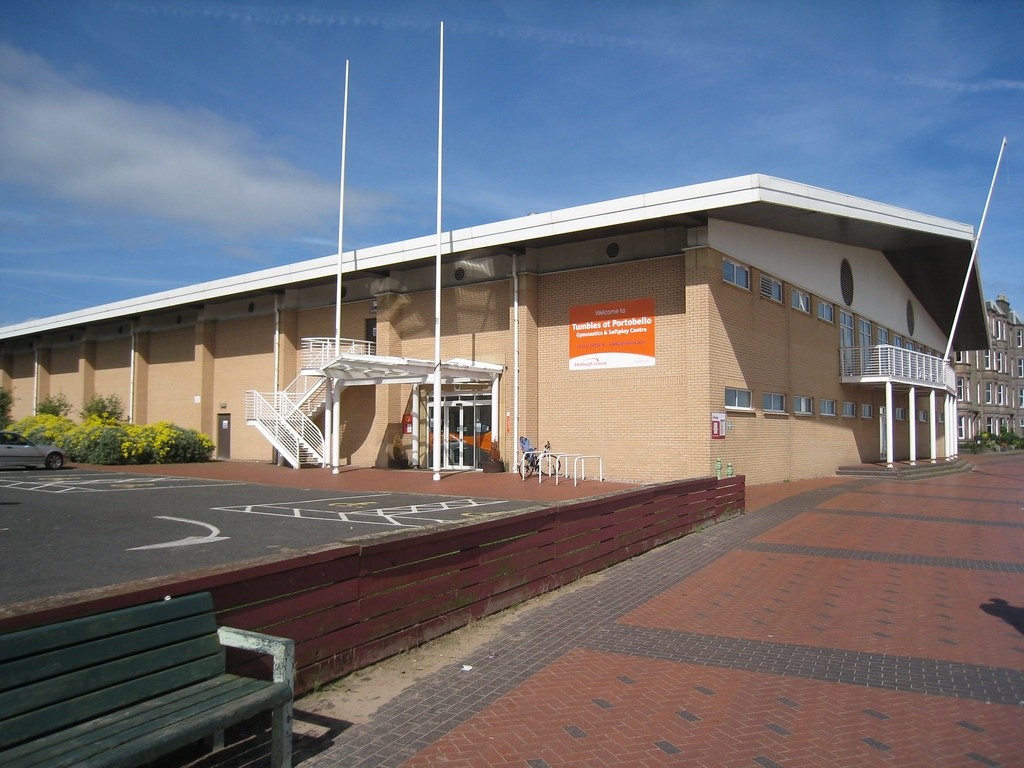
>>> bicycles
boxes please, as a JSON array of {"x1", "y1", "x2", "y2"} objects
[{"x1": 516, "y1": 441, "x2": 562, "y2": 479}]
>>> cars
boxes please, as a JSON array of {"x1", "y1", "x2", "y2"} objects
[
  {"x1": 428, "y1": 416, "x2": 492, "y2": 468},
  {"x1": 0, "y1": 431, "x2": 68, "y2": 471}
]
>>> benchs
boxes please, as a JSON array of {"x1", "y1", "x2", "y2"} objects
[{"x1": 0, "y1": 591, "x2": 294, "y2": 768}]
